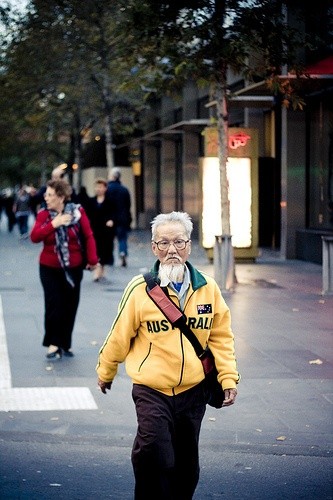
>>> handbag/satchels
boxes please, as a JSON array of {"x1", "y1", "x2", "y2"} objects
[
  {"x1": 201, "y1": 348, "x2": 225, "y2": 409},
  {"x1": 12, "y1": 203, "x2": 17, "y2": 212}
]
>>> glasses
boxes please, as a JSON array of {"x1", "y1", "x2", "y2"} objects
[
  {"x1": 43, "y1": 192, "x2": 59, "y2": 198},
  {"x1": 153, "y1": 239, "x2": 190, "y2": 251}
]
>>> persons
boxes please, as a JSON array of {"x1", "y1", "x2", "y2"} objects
[
  {"x1": 0, "y1": 167, "x2": 132, "y2": 282},
  {"x1": 95, "y1": 211, "x2": 241, "y2": 500},
  {"x1": 31, "y1": 182, "x2": 100, "y2": 362}
]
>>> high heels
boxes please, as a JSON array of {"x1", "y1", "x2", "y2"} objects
[
  {"x1": 46, "y1": 348, "x2": 62, "y2": 359},
  {"x1": 62, "y1": 348, "x2": 74, "y2": 357}
]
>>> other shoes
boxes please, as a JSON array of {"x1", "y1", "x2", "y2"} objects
[
  {"x1": 93, "y1": 276, "x2": 99, "y2": 282},
  {"x1": 120, "y1": 255, "x2": 127, "y2": 267},
  {"x1": 19, "y1": 233, "x2": 29, "y2": 240}
]
